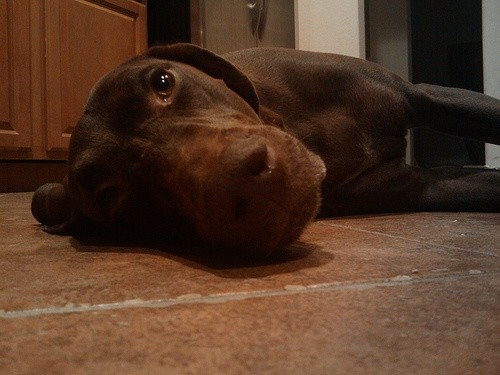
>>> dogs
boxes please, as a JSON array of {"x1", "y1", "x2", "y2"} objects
[{"x1": 30, "y1": 42, "x2": 500, "y2": 267}]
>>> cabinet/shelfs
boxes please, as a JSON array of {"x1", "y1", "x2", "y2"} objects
[{"x1": 0, "y1": 0, "x2": 152, "y2": 196}]
